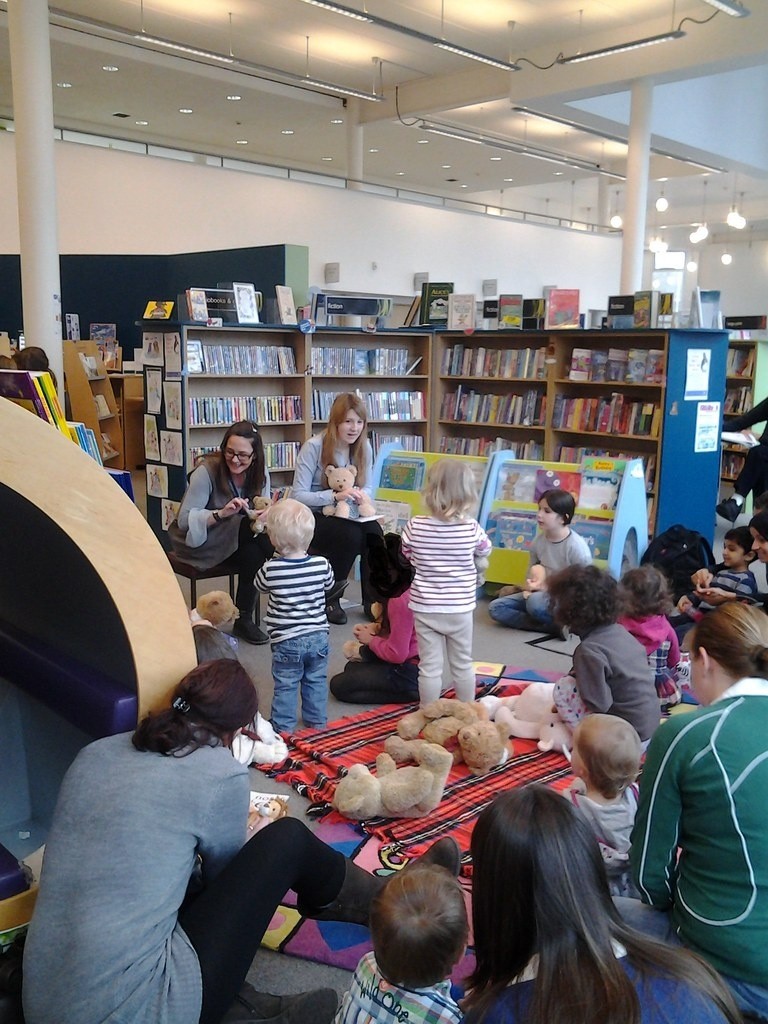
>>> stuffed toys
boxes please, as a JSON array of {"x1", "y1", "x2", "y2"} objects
[
  {"x1": 187, "y1": 589, "x2": 240, "y2": 633},
  {"x1": 245, "y1": 797, "x2": 286, "y2": 829},
  {"x1": 330, "y1": 681, "x2": 579, "y2": 820},
  {"x1": 230, "y1": 709, "x2": 290, "y2": 768},
  {"x1": 341, "y1": 602, "x2": 390, "y2": 660}
]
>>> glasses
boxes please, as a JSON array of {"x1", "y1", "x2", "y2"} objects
[{"x1": 221, "y1": 446, "x2": 254, "y2": 461}]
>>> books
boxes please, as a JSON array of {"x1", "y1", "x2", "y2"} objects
[
  {"x1": 247, "y1": 791, "x2": 289, "y2": 817},
  {"x1": 1, "y1": 280, "x2": 768, "y2": 572}
]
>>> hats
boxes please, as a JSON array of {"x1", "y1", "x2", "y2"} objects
[{"x1": 750, "y1": 509, "x2": 768, "y2": 544}]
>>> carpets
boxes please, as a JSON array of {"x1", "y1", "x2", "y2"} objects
[
  {"x1": 252, "y1": 681, "x2": 647, "y2": 883},
  {"x1": 257, "y1": 658, "x2": 704, "y2": 1008}
]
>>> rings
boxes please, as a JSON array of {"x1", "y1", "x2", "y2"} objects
[{"x1": 249, "y1": 825, "x2": 253, "y2": 831}]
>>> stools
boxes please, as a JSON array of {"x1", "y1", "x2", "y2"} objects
[{"x1": 165, "y1": 551, "x2": 262, "y2": 626}]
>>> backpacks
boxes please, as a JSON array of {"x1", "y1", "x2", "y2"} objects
[{"x1": 640, "y1": 524, "x2": 717, "y2": 575}]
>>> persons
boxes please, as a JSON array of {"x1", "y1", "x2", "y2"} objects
[
  {"x1": 626, "y1": 598, "x2": 768, "y2": 1024},
  {"x1": 289, "y1": 391, "x2": 391, "y2": 624},
  {"x1": 20, "y1": 660, "x2": 465, "y2": 1024},
  {"x1": 251, "y1": 496, "x2": 336, "y2": 733},
  {"x1": 331, "y1": 534, "x2": 425, "y2": 704},
  {"x1": 332, "y1": 863, "x2": 469, "y2": 1024},
  {"x1": 398, "y1": 458, "x2": 492, "y2": 706},
  {"x1": 453, "y1": 783, "x2": 747, "y2": 1024},
  {"x1": 545, "y1": 564, "x2": 658, "y2": 758},
  {"x1": 666, "y1": 525, "x2": 760, "y2": 644},
  {"x1": 560, "y1": 714, "x2": 648, "y2": 899},
  {"x1": 616, "y1": 565, "x2": 684, "y2": 711},
  {"x1": 486, "y1": 488, "x2": 594, "y2": 641},
  {"x1": 165, "y1": 420, "x2": 276, "y2": 647},
  {"x1": 688, "y1": 509, "x2": 768, "y2": 608}
]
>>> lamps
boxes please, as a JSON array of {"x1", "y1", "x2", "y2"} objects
[{"x1": 49, "y1": 0, "x2": 749, "y2": 269}]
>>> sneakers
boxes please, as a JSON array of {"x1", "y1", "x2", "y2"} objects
[
  {"x1": 716, "y1": 498, "x2": 743, "y2": 521},
  {"x1": 328, "y1": 596, "x2": 348, "y2": 625}
]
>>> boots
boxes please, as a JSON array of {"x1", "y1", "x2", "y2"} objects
[
  {"x1": 218, "y1": 979, "x2": 338, "y2": 1024},
  {"x1": 296, "y1": 835, "x2": 461, "y2": 928},
  {"x1": 519, "y1": 615, "x2": 570, "y2": 641},
  {"x1": 232, "y1": 603, "x2": 269, "y2": 644},
  {"x1": 324, "y1": 579, "x2": 348, "y2": 599}
]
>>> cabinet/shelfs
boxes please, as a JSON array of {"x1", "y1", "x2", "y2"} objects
[{"x1": 63, "y1": 323, "x2": 768, "y2": 598}]
